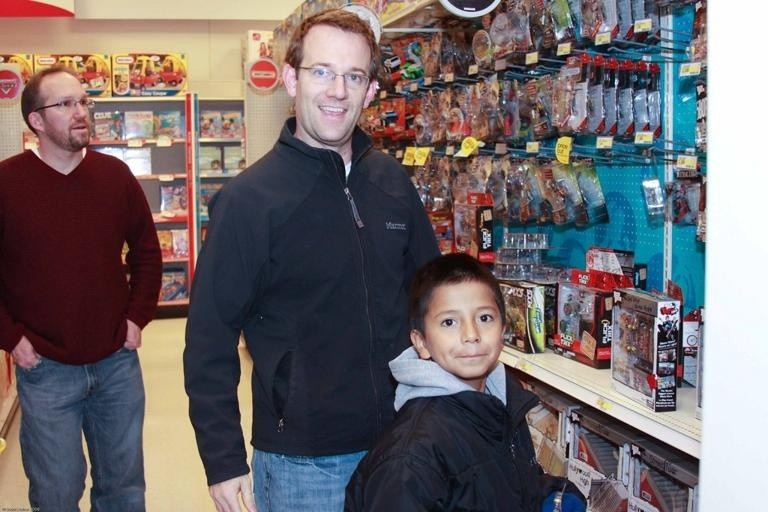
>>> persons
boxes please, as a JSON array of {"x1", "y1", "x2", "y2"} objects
[
  {"x1": 178, "y1": 7, "x2": 443, "y2": 512},
  {"x1": 341, "y1": 250, "x2": 589, "y2": 512},
  {"x1": 0, "y1": 63, "x2": 166, "y2": 512}
]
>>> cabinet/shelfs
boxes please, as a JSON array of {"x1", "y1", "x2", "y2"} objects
[{"x1": 88, "y1": 95, "x2": 245, "y2": 306}]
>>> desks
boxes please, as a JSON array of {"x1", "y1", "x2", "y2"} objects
[{"x1": 499, "y1": 341, "x2": 704, "y2": 512}]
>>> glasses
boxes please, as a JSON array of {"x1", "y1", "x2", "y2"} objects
[
  {"x1": 33, "y1": 99, "x2": 91, "y2": 113},
  {"x1": 298, "y1": 66, "x2": 366, "y2": 86}
]
